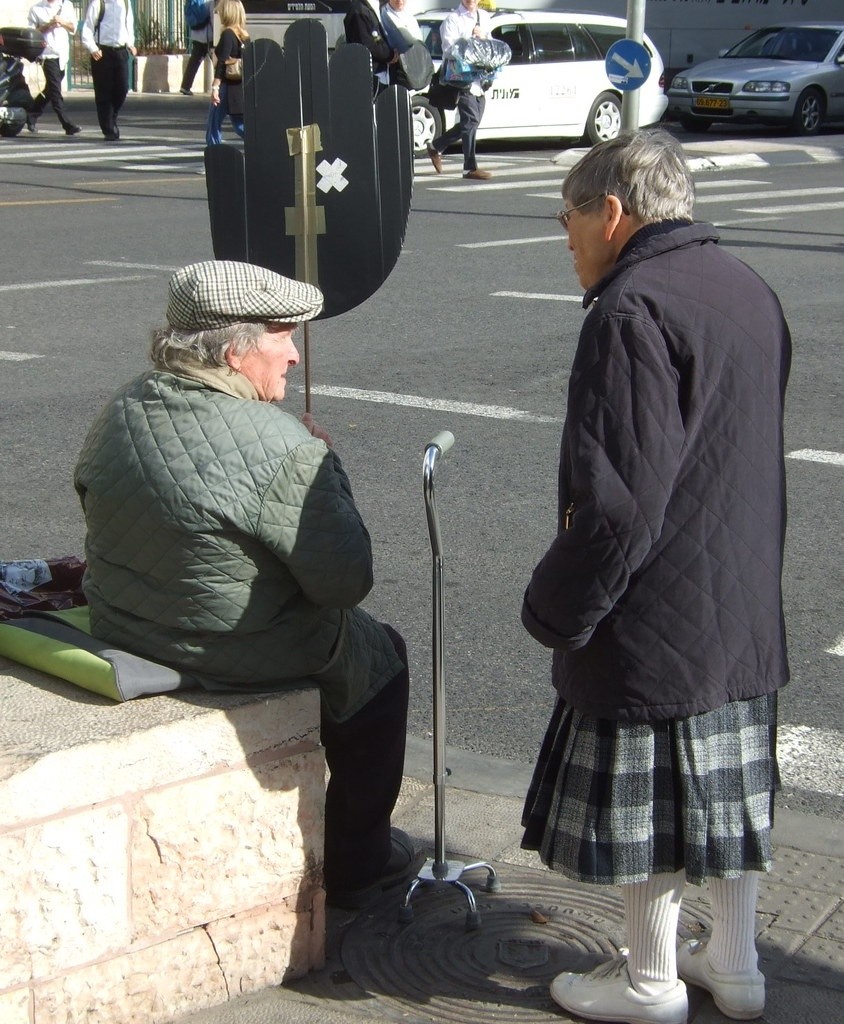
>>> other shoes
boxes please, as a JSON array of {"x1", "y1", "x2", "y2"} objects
[
  {"x1": 26, "y1": 121, "x2": 38, "y2": 132},
  {"x1": 326, "y1": 827, "x2": 414, "y2": 909},
  {"x1": 104, "y1": 131, "x2": 115, "y2": 141},
  {"x1": 66, "y1": 125, "x2": 82, "y2": 135},
  {"x1": 113, "y1": 125, "x2": 119, "y2": 138},
  {"x1": 180, "y1": 88, "x2": 193, "y2": 95}
]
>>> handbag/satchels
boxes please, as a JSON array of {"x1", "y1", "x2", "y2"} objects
[
  {"x1": 223, "y1": 28, "x2": 244, "y2": 80},
  {"x1": 428, "y1": 64, "x2": 461, "y2": 111}
]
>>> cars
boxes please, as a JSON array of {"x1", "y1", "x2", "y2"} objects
[{"x1": 667, "y1": 20, "x2": 844, "y2": 137}]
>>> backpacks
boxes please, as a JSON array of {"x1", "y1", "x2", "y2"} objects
[{"x1": 184, "y1": 0, "x2": 210, "y2": 30}]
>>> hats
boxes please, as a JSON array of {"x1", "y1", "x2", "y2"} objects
[{"x1": 166, "y1": 261, "x2": 324, "y2": 332}]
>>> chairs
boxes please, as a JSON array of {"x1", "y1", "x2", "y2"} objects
[
  {"x1": 500, "y1": 31, "x2": 523, "y2": 56},
  {"x1": 761, "y1": 35, "x2": 794, "y2": 59}
]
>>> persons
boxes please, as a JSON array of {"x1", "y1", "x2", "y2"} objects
[
  {"x1": 180, "y1": 0, "x2": 214, "y2": 95},
  {"x1": 25, "y1": 0, "x2": 137, "y2": 141},
  {"x1": 521, "y1": 128, "x2": 790, "y2": 1024},
  {"x1": 194, "y1": 0, "x2": 250, "y2": 175},
  {"x1": 73, "y1": 259, "x2": 417, "y2": 911},
  {"x1": 342, "y1": 0, "x2": 492, "y2": 181}
]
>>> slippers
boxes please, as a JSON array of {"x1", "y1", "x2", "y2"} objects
[
  {"x1": 427, "y1": 143, "x2": 442, "y2": 174},
  {"x1": 463, "y1": 169, "x2": 490, "y2": 179}
]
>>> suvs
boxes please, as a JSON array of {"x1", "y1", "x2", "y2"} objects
[{"x1": 410, "y1": 0, "x2": 669, "y2": 159}]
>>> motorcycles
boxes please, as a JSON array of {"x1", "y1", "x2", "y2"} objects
[{"x1": 0, "y1": 27, "x2": 46, "y2": 136}]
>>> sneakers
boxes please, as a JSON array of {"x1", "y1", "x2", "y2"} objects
[
  {"x1": 549, "y1": 948, "x2": 688, "y2": 1024},
  {"x1": 677, "y1": 939, "x2": 765, "y2": 1020}
]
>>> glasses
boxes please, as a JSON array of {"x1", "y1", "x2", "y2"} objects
[{"x1": 557, "y1": 193, "x2": 630, "y2": 230}]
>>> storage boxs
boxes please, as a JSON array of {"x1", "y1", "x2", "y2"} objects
[{"x1": 0, "y1": 27, "x2": 47, "y2": 62}]
